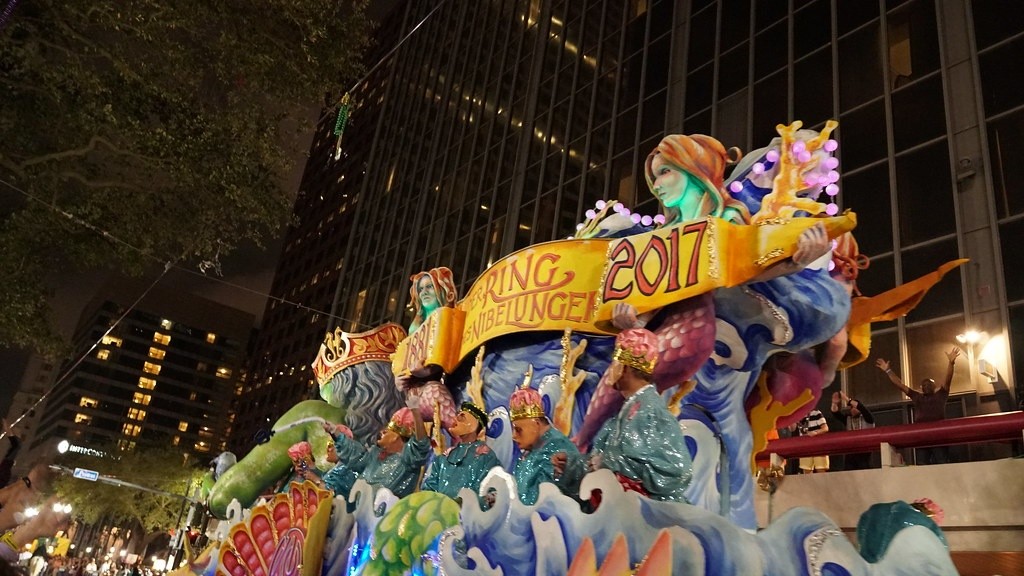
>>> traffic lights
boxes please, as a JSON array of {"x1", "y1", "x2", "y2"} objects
[{"x1": 173, "y1": 528, "x2": 184, "y2": 549}]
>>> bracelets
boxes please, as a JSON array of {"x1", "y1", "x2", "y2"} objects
[
  {"x1": 886, "y1": 369, "x2": 891, "y2": 373},
  {"x1": 848, "y1": 398, "x2": 852, "y2": 406},
  {"x1": 22, "y1": 476, "x2": 31, "y2": 488},
  {"x1": 331, "y1": 426, "x2": 336, "y2": 434},
  {"x1": 815, "y1": 430, "x2": 818, "y2": 435},
  {"x1": 0, "y1": 531, "x2": 20, "y2": 554}
]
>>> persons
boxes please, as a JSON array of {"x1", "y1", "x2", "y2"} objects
[
  {"x1": 66, "y1": 556, "x2": 178, "y2": 576},
  {"x1": 788, "y1": 409, "x2": 830, "y2": 474},
  {"x1": 288, "y1": 389, "x2": 587, "y2": 513},
  {"x1": 831, "y1": 391, "x2": 876, "y2": 471},
  {"x1": 569, "y1": 135, "x2": 834, "y2": 458},
  {"x1": 0, "y1": 418, "x2": 69, "y2": 576},
  {"x1": 551, "y1": 329, "x2": 693, "y2": 515},
  {"x1": 395, "y1": 267, "x2": 457, "y2": 447},
  {"x1": 876, "y1": 347, "x2": 960, "y2": 466}
]
